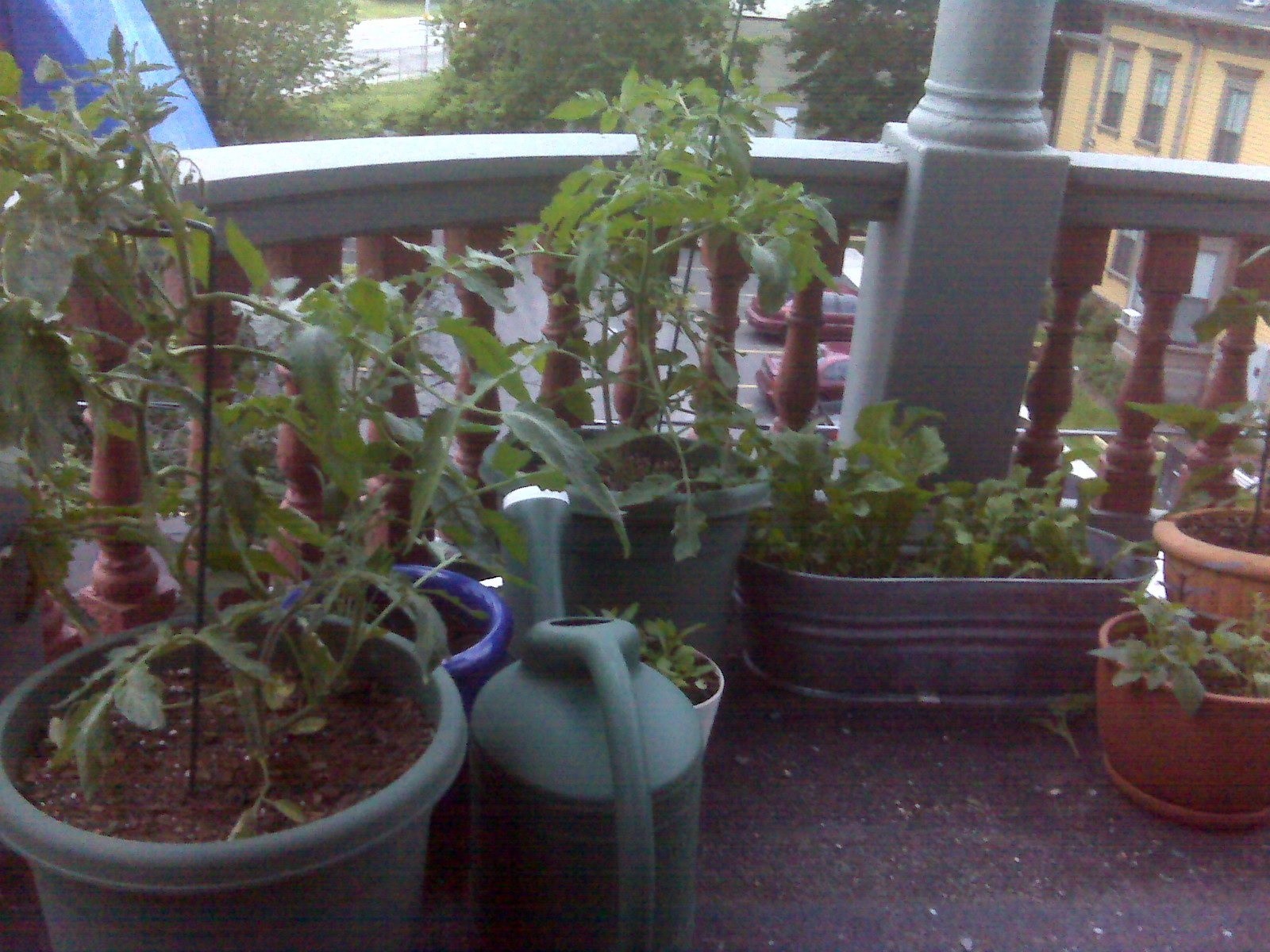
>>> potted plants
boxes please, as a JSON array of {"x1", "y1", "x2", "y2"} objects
[{"x1": 0, "y1": 19, "x2": 1270, "y2": 952}]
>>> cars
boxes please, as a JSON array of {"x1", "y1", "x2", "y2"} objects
[
  {"x1": 745, "y1": 282, "x2": 859, "y2": 339},
  {"x1": 756, "y1": 341, "x2": 852, "y2": 410}
]
